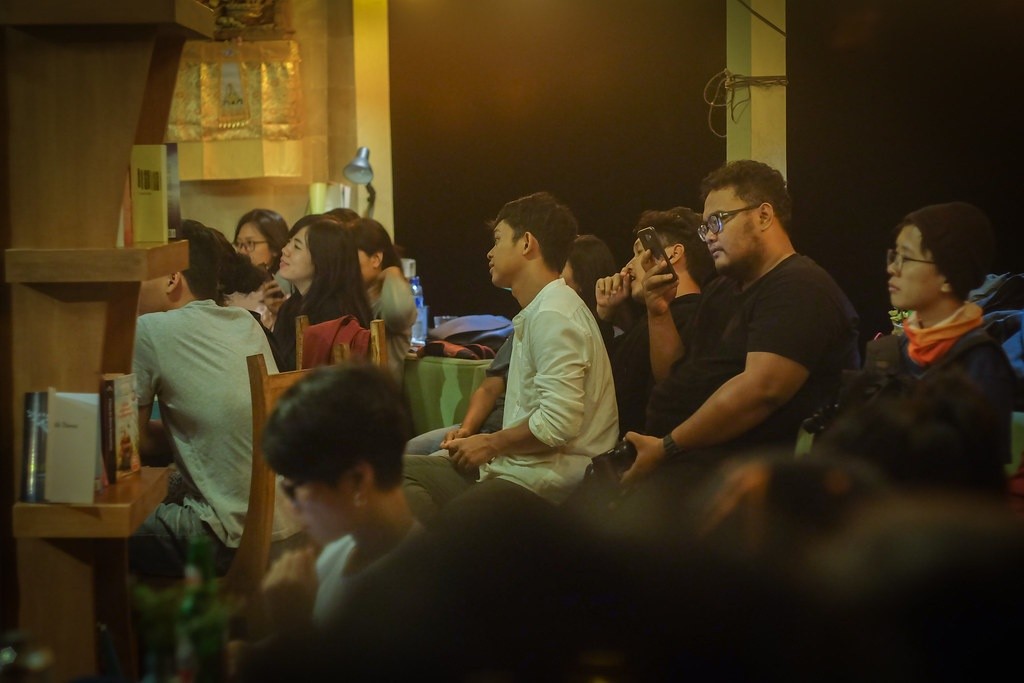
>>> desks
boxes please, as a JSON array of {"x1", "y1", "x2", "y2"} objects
[{"x1": 405, "y1": 357, "x2": 497, "y2": 438}]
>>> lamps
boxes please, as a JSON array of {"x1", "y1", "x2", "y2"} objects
[{"x1": 344, "y1": 146, "x2": 376, "y2": 207}]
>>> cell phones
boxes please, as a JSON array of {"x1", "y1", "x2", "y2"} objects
[
  {"x1": 637, "y1": 226, "x2": 678, "y2": 284},
  {"x1": 256, "y1": 262, "x2": 284, "y2": 299}
]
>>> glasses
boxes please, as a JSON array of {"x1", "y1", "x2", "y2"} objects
[
  {"x1": 886, "y1": 249, "x2": 939, "y2": 274},
  {"x1": 231, "y1": 240, "x2": 272, "y2": 253},
  {"x1": 280, "y1": 475, "x2": 316, "y2": 502},
  {"x1": 697, "y1": 206, "x2": 759, "y2": 241}
]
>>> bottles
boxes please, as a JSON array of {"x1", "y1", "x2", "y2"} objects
[
  {"x1": 177, "y1": 536, "x2": 229, "y2": 683},
  {"x1": 410, "y1": 277, "x2": 423, "y2": 310}
]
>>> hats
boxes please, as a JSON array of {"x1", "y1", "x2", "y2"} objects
[{"x1": 904, "y1": 200, "x2": 995, "y2": 292}]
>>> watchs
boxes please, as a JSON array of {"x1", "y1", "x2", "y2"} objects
[{"x1": 663, "y1": 434, "x2": 686, "y2": 463}]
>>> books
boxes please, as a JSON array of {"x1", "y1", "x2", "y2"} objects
[
  {"x1": 99, "y1": 373, "x2": 142, "y2": 482},
  {"x1": 21, "y1": 391, "x2": 101, "y2": 504}
]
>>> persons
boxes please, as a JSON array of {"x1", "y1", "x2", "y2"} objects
[
  {"x1": 394, "y1": 193, "x2": 619, "y2": 564},
  {"x1": 586, "y1": 205, "x2": 718, "y2": 436},
  {"x1": 773, "y1": 193, "x2": 1013, "y2": 500},
  {"x1": 224, "y1": 360, "x2": 585, "y2": 682},
  {"x1": 601, "y1": 157, "x2": 862, "y2": 499},
  {"x1": 122, "y1": 219, "x2": 310, "y2": 587},
  {"x1": 272, "y1": 213, "x2": 374, "y2": 374},
  {"x1": 330, "y1": 207, "x2": 418, "y2": 391},
  {"x1": 230, "y1": 208, "x2": 289, "y2": 278}
]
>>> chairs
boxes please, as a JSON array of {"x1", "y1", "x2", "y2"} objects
[{"x1": 204, "y1": 314, "x2": 402, "y2": 614}]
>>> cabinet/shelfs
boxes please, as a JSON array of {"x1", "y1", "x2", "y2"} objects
[{"x1": 0, "y1": 0, "x2": 224, "y2": 683}]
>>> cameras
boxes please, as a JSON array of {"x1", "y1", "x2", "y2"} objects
[{"x1": 590, "y1": 441, "x2": 637, "y2": 483}]
